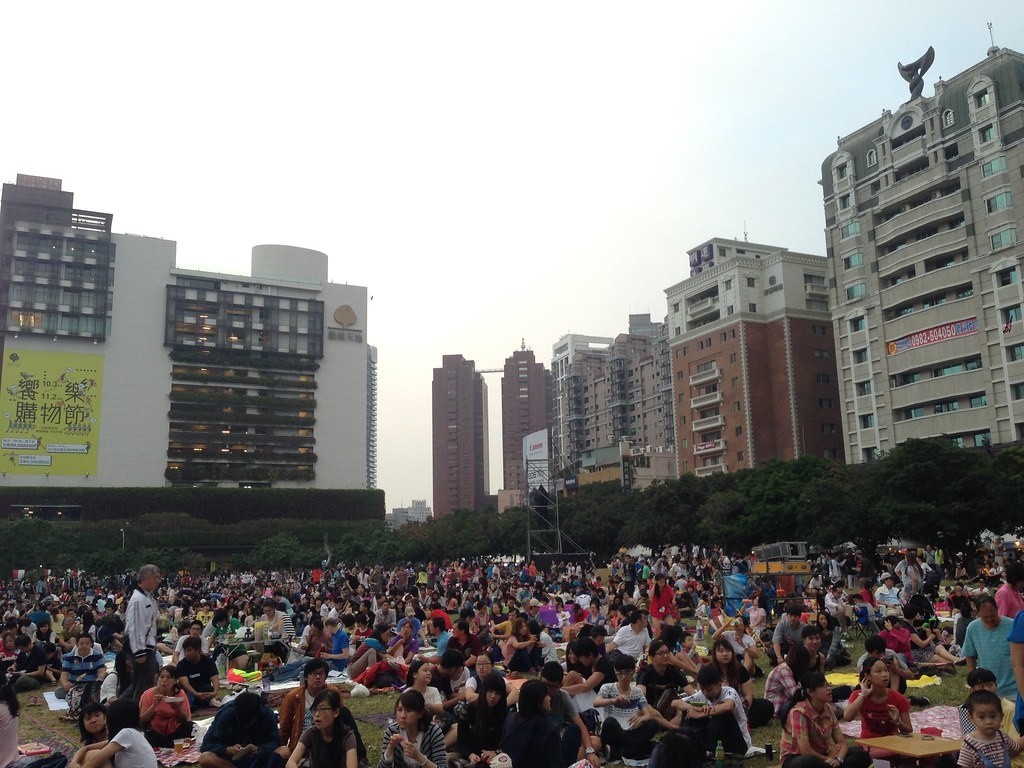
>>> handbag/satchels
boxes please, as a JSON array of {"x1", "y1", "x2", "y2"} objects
[
  {"x1": 485, "y1": 638, "x2": 505, "y2": 664},
  {"x1": 374, "y1": 670, "x2": 407, "y2": 691},
  {"x1": 24, "y1": 750, "x2": 68, "y2": 768},
  {"x1": 745, "y1": 697, "x2": 774, "y2": 729}
]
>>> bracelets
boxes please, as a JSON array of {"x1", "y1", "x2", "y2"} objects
[
  {"x1": 893, "y1": 717, "x2": 901, "y2": 725},
  {"x1": 778, "y1": 656, "x2": 781, "y2": 657},
  {"x1": 858, "y1": 693, "x2": 865, "y2": 699},
  {"x1": 424, "y1": 639, "x2": 428, "y2": 642},
  {"x1": 834, "y1": 756, "x2": 844, "y2": 764},
  {"x1": 419, "y1": 755, "x2": 427, "y2": 766},
  {"x1": 152, "y1": 704, "x2": 155, "y2": 707}
]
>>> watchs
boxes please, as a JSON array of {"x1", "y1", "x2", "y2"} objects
[
  {"x1": 457, "y1": 673, "x2": 508, "y2": 768},
  {"x1": 496, "y1": 749, "x2": 500, "y2": 755},
  {"x1": 585, "y1": 747, "x2": 596, "y2": 754}
]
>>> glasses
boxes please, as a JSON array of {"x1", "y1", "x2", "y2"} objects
[
  {"x1": 615, "y1": 669, "x2": 636, "y2": 676},
  {"x1": 311, "y1": 707, "x2": 336, "y2": 715},
  {"x1": 309, "y1": 670, "x2": 327, "y2": 678},
  {"x1": 652, "y1": 650, "x2": 671, "y2": 655},
  {"x1": 476, "y1": 662, "x2": 492, "y2": 668},
  {"x1": 262, "y1": 609, "x2": 272, "y2": 613}
]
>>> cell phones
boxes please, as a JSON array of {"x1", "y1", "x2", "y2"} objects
[
  {"x1": 690, "y1": 701, "x2": 709, "y2": 707},
  {"x1": 535, "y1": 635, "x2": 540, "y2": 642},
  {"x1": 240, "y1": 747, "x2": 248, "y2": 751}
]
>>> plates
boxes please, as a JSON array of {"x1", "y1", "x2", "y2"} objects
[{"x1": 163, "y1": 696, "x2": 184, "y2": 702}]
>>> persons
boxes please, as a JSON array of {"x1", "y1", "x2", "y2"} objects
[
  {"x1": 285, "y1": 688, "x2": 357, "y2": 768},
  {"x1": 670, "y1": 663, "x2": 751, "y2": 764},
  {"x1": 501, "y1": 679, "x2": 582, "y2": 768},
  {"x1": 780, "y1": 670, "x2": 876, "y2": 768},
  {"x1": 592, "y1": 655, "x2": 658, "y2": 759},
  {"x1": 502, "y1": 618, "x2": 545, "y2": 673},
  {"x1": 0, "y1": 537, "x2": 1024, "y2": 768},
  {"x1": 844, "y1": 656, "x2": 919, "y2": 768},
  {"x1": 376, "y1": 690, "x2": 447, "y2": 768},
  {"x1": 960, "y1": 595, "x2": 1018, "y2": 703},
  {"x1": 958, "y1": 689, "x2": 1024, "y2": 768}
]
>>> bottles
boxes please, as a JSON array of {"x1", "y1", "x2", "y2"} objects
[
  {"x1": 742, "y1": 599, "x2": 754, "y2": 604},
  {"x1": 263, "y1": 675, "x2": 270, "y2": 693},
  {"x1": 765, "y1": 734, "x2": 774, "y2": 761},
  {"x1": 642, "y1": 654, "x2": 648, "y2": 668},
  {"x1": 219, "y1": 627, "x2": 225, "y2": 643},
  {"x1": 696, "y1": 619, "x2": 704, "y2": 639},
  {"x1": 715, "y1": 741, "x2": 726, "y2": 768}
]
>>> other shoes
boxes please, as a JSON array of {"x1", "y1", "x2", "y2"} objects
[
  {"x1": 59, "y1": 716, "x2": 75, "y2": 724},
  {"x1": 27, "y1": 695, "x2": 44, "y2": 706}
]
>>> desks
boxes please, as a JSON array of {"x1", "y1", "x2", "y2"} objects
[
  {"x1": 768, "y1": 597, "x2": 818, "y2": 627},
  {"x1": 854, "y1": 732, "x2": 963, "y2": 768},
  {"x1": 215, "y1": 634, "x2": 294, "y2": 678}
]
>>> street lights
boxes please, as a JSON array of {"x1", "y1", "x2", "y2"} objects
[{"x1": 120, "y1": 529, "x2": 125, "y2": 549}]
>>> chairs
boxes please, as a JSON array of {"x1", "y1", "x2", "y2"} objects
[
  {"x1": 816, "y1": 593, "x2": 869, "y2": 639},
  {"x1": 857, "y1": 602, "x2": 887, "y2": 636}
]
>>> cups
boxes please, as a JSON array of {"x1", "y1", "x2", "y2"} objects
[
  {"x1": 173, "y1": 739, "x2": 184, "y2": 755},
  {"x1": 730, "y1": 619, "x2": 741, "y2": 627}
]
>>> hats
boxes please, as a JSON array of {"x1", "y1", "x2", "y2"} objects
[
  {"x1": 880, "y1": 572, "x2": 892, "y2": 581},
  {"x1": 405, "y1": 606, "x2": 416, "y2": 616},
  {"x1": 390, "y1": 627, "x2": 398, "y2": 634},
  {"x1": 294, "y1": 591, "x2": 301, "y2": 598},
  {"x1": 200, "y1": 598, "x2": 207, "y2": 604},
  {"x1": 299, "y1": 593, "x2": 306, "y2": 605},
  {"x1": 846, "y1": 548, "x2": 854, "y2": 553},
  {"x1": 530, "y1": 598, "x2": 544, "y2": 607},
  {"x1": 855, "y1": 550, "x2": 863, "y2": 558},
  {"x1": 977, "y1": 578, "x2": 987, "y2": 583}
]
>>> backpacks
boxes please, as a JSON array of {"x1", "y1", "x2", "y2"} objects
[
  {"x1": 79, "y1": 672, "x2": 119, "y2": 709},
  {"x1": 64, "y1": 684, "x2": 86, "y2": 717},
  {"x1": 646, "y1": 728, "x2": 709, "y2": 768}
]
